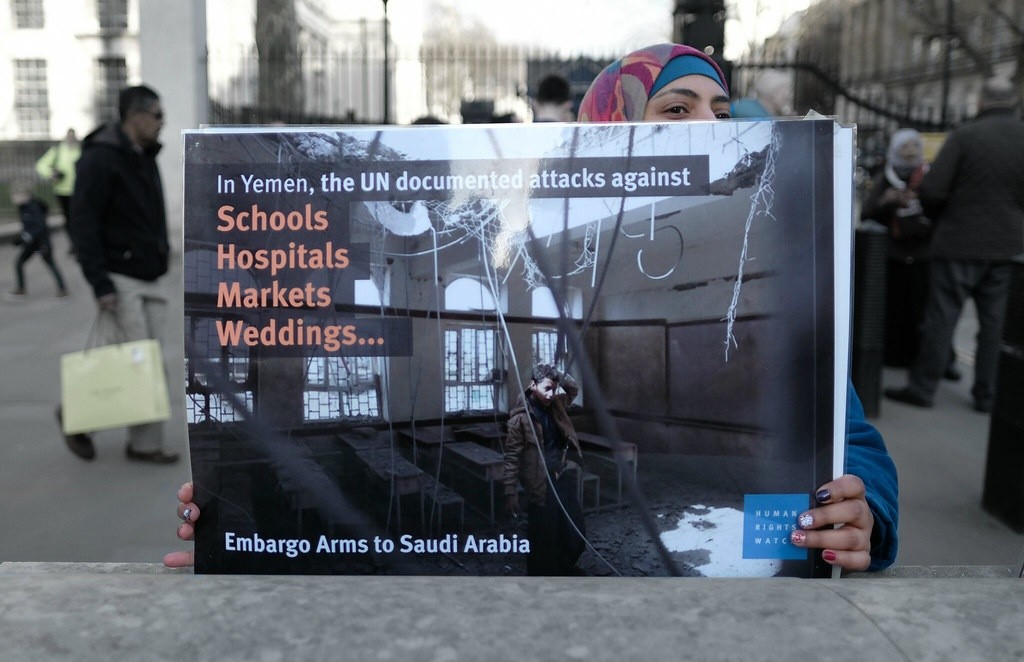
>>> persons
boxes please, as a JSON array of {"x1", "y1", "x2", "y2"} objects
[
  {"x1": 503, "y1": 362, "x2": 586, "y2": 576},
  {"x1": 860, "y1": 75, "x2": 1024, "y2": 415},
  {"x1": 36, "y1": 127, "x2": 83, "y2": 254},
  {"x1": 163, "y1": 41, "x2": 900, "y2": 571},
  {"x1": 531, "y1": 73, "x2": 571, "y2": 122},
  {"x1": 55, "y1": 85, "x2": 181, "y2": 467},
  {"x1": 10, "y1": 180, "x2": 69, "y2": 300},
  {"x1": 458, "y1": 99, "x2": 515, "y2": 124}
]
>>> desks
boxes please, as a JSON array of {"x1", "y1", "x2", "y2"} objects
[{"x1": 269, "y1": 397, "x2": 637, "y2": 562}]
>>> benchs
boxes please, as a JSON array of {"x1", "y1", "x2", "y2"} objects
[
  {"x1": 582, "y1": 471, "x2": 600, "y2": 516},
  {"x1": 420, "y1": 469, "x2": 466, "y2": 532}
]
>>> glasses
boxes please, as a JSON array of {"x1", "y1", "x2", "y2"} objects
[{"x1": 136, "y1": 109, "x2": 162, "y2": 119}]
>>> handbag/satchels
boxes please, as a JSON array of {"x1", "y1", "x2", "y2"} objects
[
  {"x1": 51, "y1": 146, "x2": 64, "y2": 183},
  {"x1": 60, "y1": 305, "x2": 171, "y2": 436}
]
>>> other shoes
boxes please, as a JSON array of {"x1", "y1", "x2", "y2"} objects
[
  {"x1": 126, "y1": 442, "x2": 179, "y2": 464},
  {"x1": 943, "y1": 367, "x2": 960, "y2": 380},
  {"x1": 976, "y1": 399, "x2": 994, "y2": 411},
  {"x1": 8, "y1": 288, "x2": 26, "y2": 296},
  {"x1": 56, "y1": 289, "x2": 69, "y2": 299},
  {"x1": 884, "y1": 387, "x2": 934, "y2": 409},
  {"x1": 56, "y1": 407, "x2": 96, "y2": 460}
]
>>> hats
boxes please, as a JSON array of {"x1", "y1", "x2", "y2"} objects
[
  {"x1": 648, "y1": 55, "x2": 729, "y2": 100},
  {"x1": 980, "y1": 76, "x2": 1019, "y2": 112}
]
